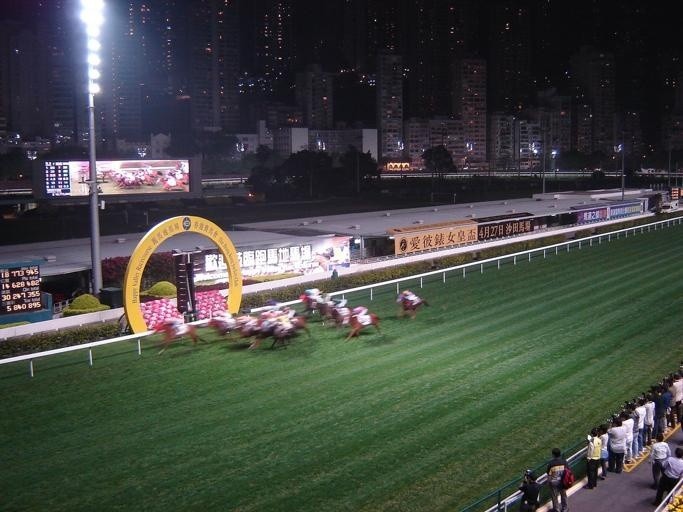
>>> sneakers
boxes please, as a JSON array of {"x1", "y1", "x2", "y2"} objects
[
  {"x1": 548, "y1": 506, "x2": 570, "y2": 512},
  {"x1": 582, "y1": 418, "x2": 683, "y2": 507}
]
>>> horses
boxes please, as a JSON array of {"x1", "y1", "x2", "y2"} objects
[
  {"x1": 153, "y1": 323, "x2": 207, "y2": 355},
  {"x1": 110, "y1": 161, "x2": 190, "y2": 192},
  {"x1": 396, "y1": 294, "x2": 431, "y2": 321},
  {"x1": 209, "y1": 293, "x2": 383, "y2": 352}
]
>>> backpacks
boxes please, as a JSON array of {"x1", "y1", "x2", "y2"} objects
[{"x1": 562, "y1": 460, "x2": 574, "y2": 490}]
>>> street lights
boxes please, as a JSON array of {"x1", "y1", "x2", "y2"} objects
[
  {"x1": 397, "y1": 139, "x2": 404, "y2": 180},
  {"x1": 318, "y1": 139, "x2": 325, "y2": 152},
  {"x1": 613, "y1": 143, "x2": 620, "y2": 177},
  {"x1": 235, "y1": 142, "x2": 247, "y2": 184},
  {"x1": 528, "y1": 142, "x2": 536, "y2": 176},
  {"x1": 136, "y1": 146, "x2": 146, "y2": 158},
  {"x1": 26, "y1": 148, "x2": 37, "y2": 189},
  {"x1": 76, "y1": 0, "x2": 111, "y2": 313},
  {"x1": 466, "y1": 141, "x2": 474, "y2": 177}
]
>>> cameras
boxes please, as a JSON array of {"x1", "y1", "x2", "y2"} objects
[
  {"x1": 607, "y1": 366, "x2": 683, "y2": 421},
  {"x1": 525, "y1": 470, "x2": 531, "y2": 479}
]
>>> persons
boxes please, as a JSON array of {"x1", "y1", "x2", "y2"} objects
[
  {"x1": 106, "y1": 161, "x2": 189, "y2": 184},
  {"x1": 546, "y1": 447, "x2": 568, "y2": 512},
  {"x1": 518, "y1": 472, "x2": 541, "y2": 512},
  {"x1": 583, "y1": 362, "x2": 683, "y2": 507},
  {"x1": 164, "y1": 287, "x2": 422, "y2": 338}
]
